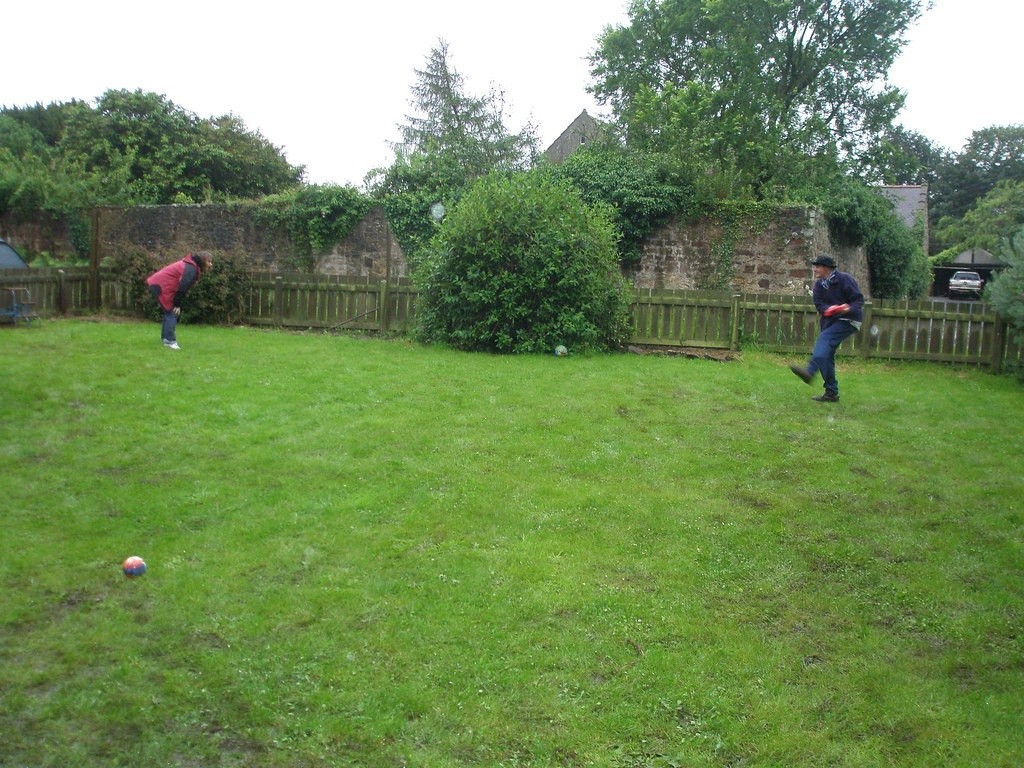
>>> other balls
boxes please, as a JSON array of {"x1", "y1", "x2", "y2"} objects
[
  {"x1": 556, "y1": 346, "x2": 567, "y2": 358},
  {"x1": 123, "y1": 555, "x2": 148, "y2": 578}
]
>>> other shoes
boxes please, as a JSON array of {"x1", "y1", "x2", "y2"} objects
[
  {"x1": 164, "y1": 341, "x2": 180, "y2": 350},
  {"x1": 810, "y1": 394, "x2": 839, "y2": 402},
  {"x1": 789, "y1": 364, "x2": 812, "y2": 383}
]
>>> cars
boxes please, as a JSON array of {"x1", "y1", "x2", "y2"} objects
[{"x1": 947, "y1": 270, "x2": 985, "y2": 302}]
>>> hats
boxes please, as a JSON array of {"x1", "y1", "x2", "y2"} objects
[{"x1": 811, "y1": 255, "x2": 838, "y2": 268}]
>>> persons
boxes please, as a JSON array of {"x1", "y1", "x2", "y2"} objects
[
  {"x1": 789, "y1": 256, "x2": 864, "y2": 402},
  {"x1": 147, "y1": 251, "x2": 215, "y2": 349}
]
